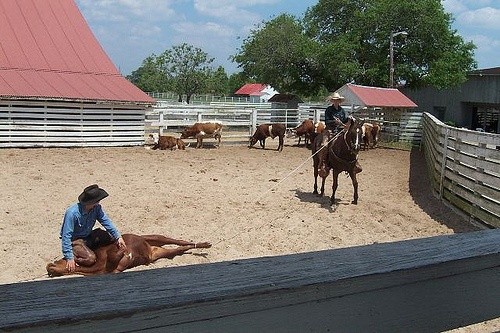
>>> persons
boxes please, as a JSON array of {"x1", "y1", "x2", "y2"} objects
[
  {"x1": 60, "y1": 184, "x2": 127, "y2": 272},
  {"x1": 319, "y1": 93, "x2": 349, "y2": 178}
]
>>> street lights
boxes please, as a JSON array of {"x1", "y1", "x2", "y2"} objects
[{"x1": 389, "y1": 31, "x2": 408, "y2": 89}]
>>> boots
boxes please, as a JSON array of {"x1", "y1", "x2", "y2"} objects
[{"x1": 317, "y1": 145, "x2": 329, "y2": 171}]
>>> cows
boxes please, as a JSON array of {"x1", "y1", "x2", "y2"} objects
[
  {"x1": 158, "y1": 137, "x2": 186, "y2": 151},
  {"x1": 46, "y1": 234, "x2": 212, "y2": 275},
  {"x1": 181, "y1": 122, "x2": 222, "y2": 149},
  {"x1": 249, "y1": 124, "x2": 286, "y2": 151},
  {"x1": 286, "y1": 119, "x2": 381, "y2": 150}
]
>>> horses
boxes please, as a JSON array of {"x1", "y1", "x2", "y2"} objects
[{"x1": 312, "y1": 117, "x2": 366, "y2": 205}]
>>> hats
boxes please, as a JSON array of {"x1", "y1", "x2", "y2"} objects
[
  {"x1": 328, "y1": 93, "x2": 345, "y2": 103},
  {"x1": 78, "y1": 184, "x2": 109, "y2": 206}
]
